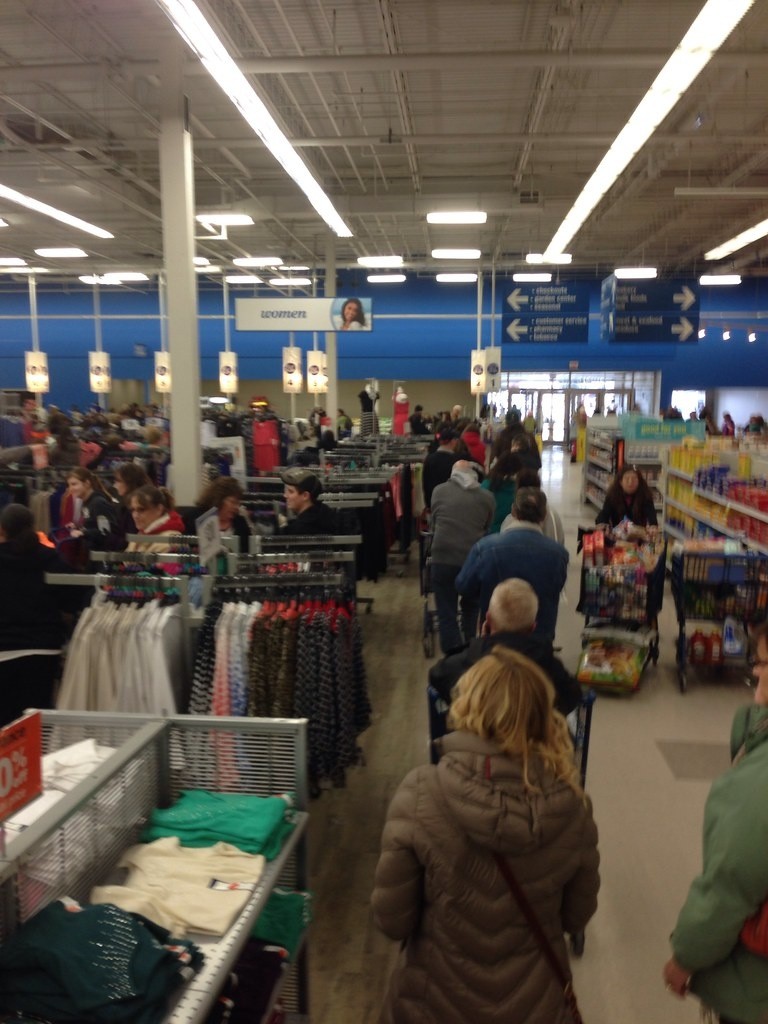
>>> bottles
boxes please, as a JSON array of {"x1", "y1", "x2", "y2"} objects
[
  {"x1": 707, "y1": 628, "x2": 724, "y2": 665},
  {"x1": 722, "y1": 615, "x2": 748, "y2": 658},
  {"x1": 626, "y1": 445, "x2": 659, "y2": 458},
  {"x1": 588, "y1": 429, "x2": 615, "y2": 504},
  {"x1": 689, "y1": 627, "x2": 706, "y2": 665}
]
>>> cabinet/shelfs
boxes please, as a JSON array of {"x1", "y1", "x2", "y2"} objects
[
  {"x1": 580, "y1": 425, "x2": 768, "y2": 573},
  {"x1": 0, "y1": 709, "x2": 309, "y2": 1024}
]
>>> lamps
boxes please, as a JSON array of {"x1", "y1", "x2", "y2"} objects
[
  {"x1": 307, "y1": 268, "x2": 329, "y2": 393},
  {"x1": 218, "y1": 277, "x2": 238, "y2": 394},
  {"x1": 89, "y1": 285, "x2": 112, "y2": 394},
  {"x1": 25, "y1": 277, "x2": 50, "y2": 394},
  {"x1": 283, "y1": 270, "x2": 304, "y2": 393},
  {"x1": 470, "y1": 254, "x2": 501, "y2": 393},
  {"x1": 154, "y1": 275, "x2": 173, "y2": 394}
]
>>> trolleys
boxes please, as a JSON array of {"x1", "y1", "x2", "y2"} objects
[
  {"x1": 418, "y1": 531, "x2": 475, "y2": 659},
  {"x1": 669, "y1": 554, "x2": 764, "y2": 695},
  {"x1": 575, "y1": 520, "x2": 669, "y2": 696}
]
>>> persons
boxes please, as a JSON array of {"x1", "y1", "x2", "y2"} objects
[
  {"x1": 410, "y1": 404, "x2": 565, "y2": 543},
  {"x1": 666, "y1": 626, "x2": 768, "y2": 1024},
  {"x1": 393, "y1": 387, "x2": 409, "y2": 435},
  {"x1": 595, "y1": 465, "x2": 659, "y2": 526},
  {"x1": 428, "y1": 577, "x2": 581, "y2": 762},
  {"x1": 339, "y1": 298, "x2": 369, "y2": 330},
  {"x1": 358, "y1": 384, "x2": 380, "y2": 434},
  {"x1": 431, "y1": 460, "x2": 496, "y2": 654},
  {"x1": 0, "y1": 505, "x2": 60, "y2": 724},
  {"x1": 372, "y1": 647, "x2": 600, "y2": 1024},
  {"x1": 45, "y1": 403, "x2": 360, "y2": 551},
  {"x1": 592, "y1": 406, "x2": 768, "y2": 444},
  {"x1": 457, "y1": 488, "x2": 570, "y2": 645}
]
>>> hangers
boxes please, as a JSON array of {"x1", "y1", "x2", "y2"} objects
[
  {"x1": 92, "y1": 535, "x2": 354, "y2": 615},
  {"x1": 242, "y1": 492, "x2": 280, "y2": 515}
]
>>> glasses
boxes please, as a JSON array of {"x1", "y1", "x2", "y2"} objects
[
  {"x1": 749, "y1": 654, "x2": 768, "y2": 668},
  {"x1": 128, "y1": 506, "x2": 156, "y2": 513}
]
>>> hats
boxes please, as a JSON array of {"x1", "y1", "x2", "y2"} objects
[
  {"x1": 439, "y1": 427, "x2": 460, "y2": 441},
  {"x1": 279, "y1": 469, "x2": 322, "y2": 499}
]
